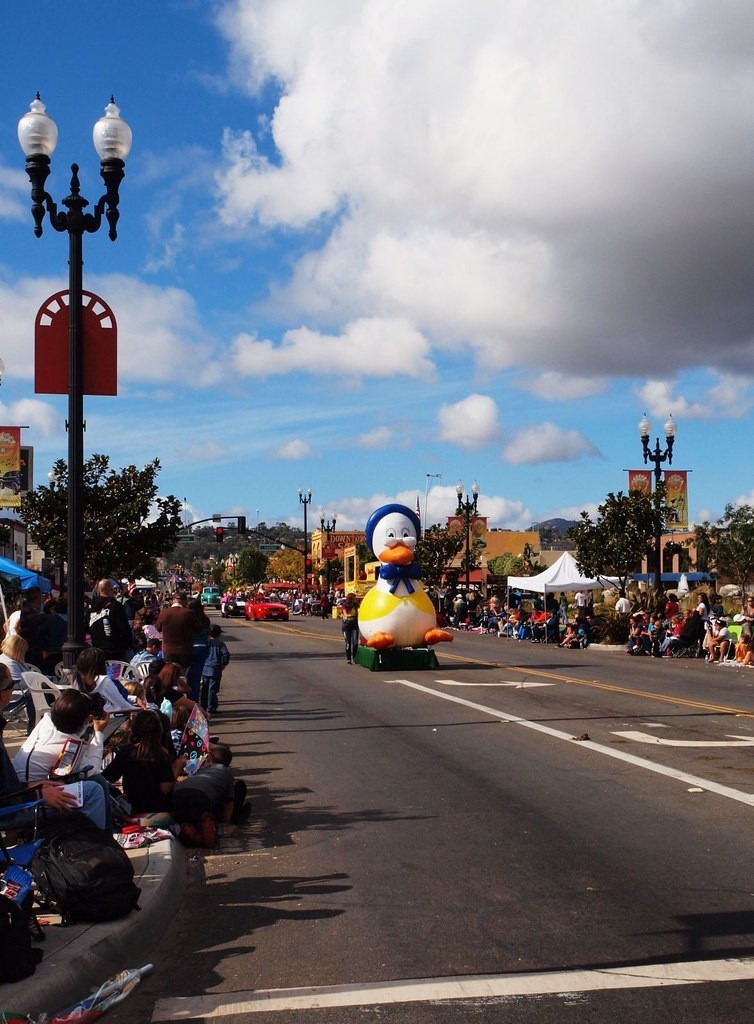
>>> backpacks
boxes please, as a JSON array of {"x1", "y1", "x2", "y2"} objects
[{"x1": 29, "y1": 809, "x2": 143, "y2": 926}]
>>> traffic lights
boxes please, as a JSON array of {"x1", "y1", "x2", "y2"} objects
[{"x1": 216, "y1": 527, "x2": 224, "y2": 542}]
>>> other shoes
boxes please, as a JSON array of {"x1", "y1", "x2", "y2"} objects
[
  {"x1": 348, "y1": 660, "x2": 351, "y2": 665},
  {"x1": 210, "y1": 710, "x2": 221, "y2": 714},
  {"x1": 234, "y1": 802, "x2": 252, "y2": 825},
  {"x1": 179, "y1": 822, "x2": 204, "y2": 848},
  {"x1": 234, "y1": 779, "x2": 247, "y2": 808},
  {"x1": 555, "y1": 645, "x2": 563, "y2": 648},
  {"x1": 200, "y1": 811, "x2": 219, "y2": 849}
]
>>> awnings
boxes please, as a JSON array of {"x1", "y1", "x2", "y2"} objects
[
  {"x1": 0, "y1": 553, "x2": 50, "y2": 601},
  {"x1": 633, "y1": 571, "x2": 718, "y2": 586}
]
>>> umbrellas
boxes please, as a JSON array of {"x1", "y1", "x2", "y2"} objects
[{"x1": 268, "y1": 581, "x2": 299, "y2": 589}]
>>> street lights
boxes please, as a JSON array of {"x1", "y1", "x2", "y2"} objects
[
  {"x1": 637, "y1": 412, "x2": 678, "y2": 588},
  {"x1": 298, "y1": 487, "x2": 312, "y2": 613},
  {"x1": 454, "y1": 478, "x2": 480, "y2": 593},
  {"x1": 16, "y1": 90, "x2": 135, "y2": 691},
  {"x1": 319, "y1": 508, "x2": 338, "y2": 594}
]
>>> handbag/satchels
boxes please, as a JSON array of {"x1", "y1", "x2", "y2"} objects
[{"x1": 0, "y1": 894, "x2": 36, "y2": 983}]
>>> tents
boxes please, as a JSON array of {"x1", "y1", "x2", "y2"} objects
[
  {"x1": 128, "y1": 576, "x2": 157, "y2": 597},
  {"x1": 507, "y1": 551, "x2": 630, "y2": 644}
]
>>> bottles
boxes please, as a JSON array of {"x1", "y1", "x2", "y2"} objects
[
  {"x1": 106, "y1": 662, "x2": 121, "y2": 680},
  {"x1": 161, "y1": 697, "x2": 172, "y2": 723},
  {"x1": 103, "y1": 618, "x2": 112, "y2": 636},
  {"x1": 156, "y1": 651, "x2": 163, "y2": 661},
  {"x1": 85, "y1": 634, "x2": 93, "y2": 646}
]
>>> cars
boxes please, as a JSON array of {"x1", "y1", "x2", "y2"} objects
[
  {"x1": 199, "y1": 585, "x2": 223, "y2": 609},
  {"x1": 244, "y1": 597, "x2": 290, "y2": 622},
  {"x1": 221, "y1": 596, "x2": 248, "y2": 619}
]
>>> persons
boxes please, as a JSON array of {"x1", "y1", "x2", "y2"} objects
[
  {"x1": 257, "y1": 584, "x2": 281, "y2": 602},
  {"x1": 0, "y1": 579, "x2": 251, "y2": 1024},
  {"x1": 342, "y1": 593, "x2": 360, "y2": 665},
  {"x1": 429, "y1": 580, "x2": 594, "y2": 649},
  {"x1": 615, "y1": 590, "x2": 633, "y2": 643},
  {"x1": 627, "y1": 592, "x2": 754, "y2": 668},
  {"x1": 282, "y1": 590, "x2": 344, "y2": 620}
]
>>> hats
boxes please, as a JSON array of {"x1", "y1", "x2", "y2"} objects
[
  {"x1": 210, "y1": 624, "x2": 223, "y2": 633},
  {"x1": 173, "y1": 593, "x2": 187, "y2": 601}
]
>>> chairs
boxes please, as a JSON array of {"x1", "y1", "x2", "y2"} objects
[
  {"x1": 709, "y1": 624, "x2": 743, "y2": 661},
  {"x1": 673, "y1": 634, "x2": 699, "y2": 658},
  {"x1": 0, "y1": 785, "x2": 54, "y2": 942},
  {"x1": 0, "y1": 660, "x2": 150, "y2": 737}
]
